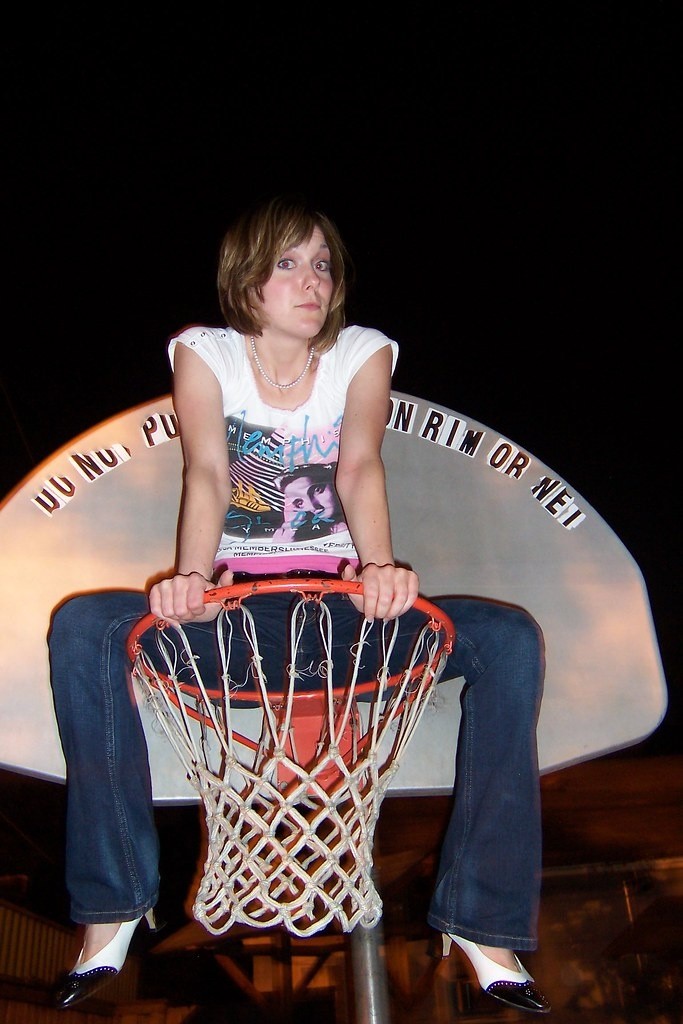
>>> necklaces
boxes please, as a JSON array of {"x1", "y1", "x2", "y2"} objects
[{"x1": 250, "y1": 332, "x2": 316, "y2": 389}]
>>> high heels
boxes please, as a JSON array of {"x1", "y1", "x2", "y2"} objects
[
  {"x1": 441, "y1": 930, "x2": 551, "y2": 1013},
  {"x1": 57, "y1": 908, "x2": 165, "y2": 1008}
]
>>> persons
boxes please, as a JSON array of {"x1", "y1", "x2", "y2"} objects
[{"x1": 44, "y1": 195, "x2": 552, "y2": 1014}]
